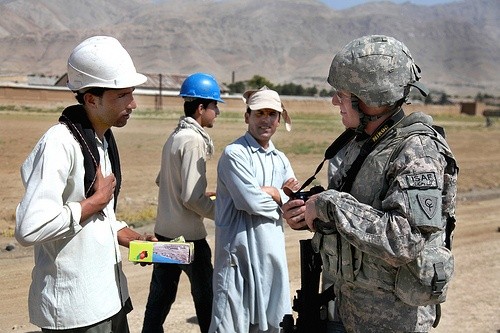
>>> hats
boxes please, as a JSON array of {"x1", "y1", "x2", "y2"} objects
[{"x1": 242, "y1": 85, "x2": 292, "y2": 132}]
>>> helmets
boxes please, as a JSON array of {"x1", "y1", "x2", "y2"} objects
[
  {"x1": 66, "y1": 35, "x2": 148, "y2": 92},
  {"x1": 178, "y1": 73, "x2": 225, "y2": 103},
  {"x1": 326, "y1": 34, "x2": 422, "y2": 108}
]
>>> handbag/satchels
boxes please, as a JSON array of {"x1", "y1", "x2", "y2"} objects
[{"x1": 394, "y1": 244, "x2": 455, "y2": 307}]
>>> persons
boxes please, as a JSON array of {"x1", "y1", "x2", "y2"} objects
[
  {"x1": 280, "y1": 35, "x2": 459, "y2": 333},
  {"x1": 205, "y1": 86, "x2": 304, "y2": 333},
  {"x1": 14, "y1": 35, "x2": 158, "y2": 333},
  {"x1": 141, "y1": 74, "x2": 224, "y2": 333}
]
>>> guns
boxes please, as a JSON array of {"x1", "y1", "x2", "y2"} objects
[{"x1": 278, "y1": 239, "x2": 336, "y2": 333}]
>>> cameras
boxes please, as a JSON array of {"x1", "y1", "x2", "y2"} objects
[{"x1": 289, "y1": 186, "x2": 340, "y2": 235}]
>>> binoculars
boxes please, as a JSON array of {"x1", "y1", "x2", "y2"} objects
[{"x1": 293, "y1": 186, "x2": 335, "y2": 235}]
